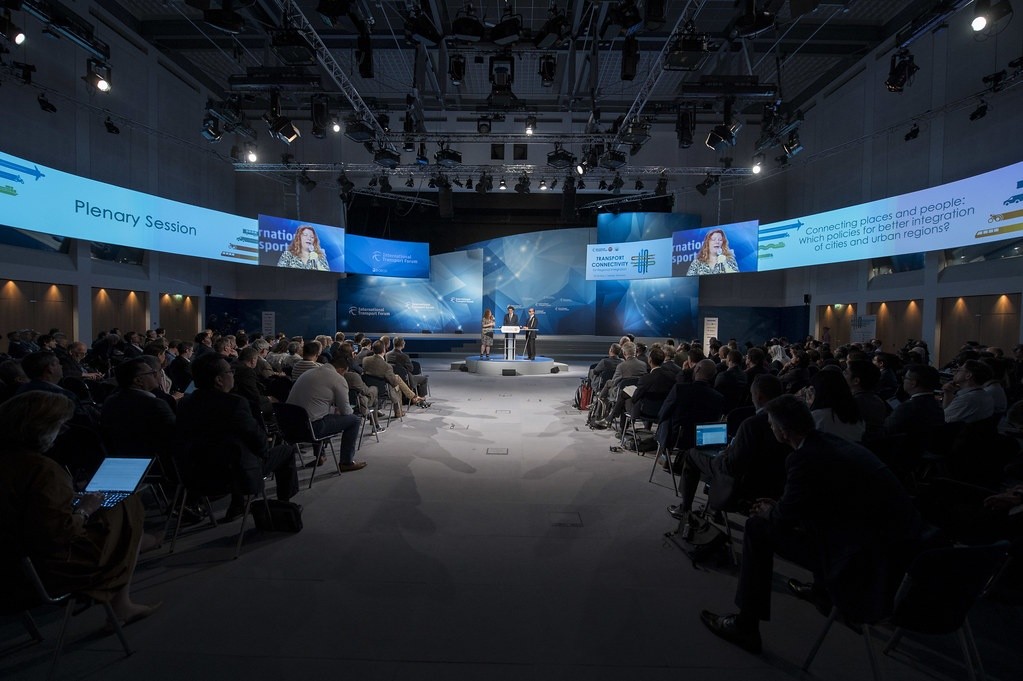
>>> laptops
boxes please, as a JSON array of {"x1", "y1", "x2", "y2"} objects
[
  {"x1": 694, "y1": 422, "x2": 728, "y2": 457},
  {"x1": 72, "y1": 456, "x2": 156, "y2": 511}
]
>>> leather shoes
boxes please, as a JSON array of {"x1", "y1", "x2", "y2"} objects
[
  {"x1": 595, "y1": 418, "x2": 612, "y2": 428},
  {"x1": 658, "y1": 455, "x2": 670, "y2": 468},
  {"x1": 700, "y1": 608, "x2": 764, "y2": 654},
  {"x1": 667, "y1": 505, "x2": 684, "y2": 519}
]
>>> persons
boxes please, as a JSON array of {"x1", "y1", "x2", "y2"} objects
[
  {"x1": 686, "y1": 229, "x2": 740, "y2": 276},
  {"x1": 277, "y1": 225, "x2": 330, "y2": 271},
  {"x1": 480, "y1": 309, "x2": 495, "y2": 360},
  {"x1": 0, "y1": 314, "x2": 433, "y2": 628},
  {"x1": 588, "y1": 327, "x2": 1023, "y2": 653},
  {"x1": 504, "y1": 306, "x2": 518, "y2": 359},
  {"x1": 523, "y1": 307, "x2": 538, "y2": 360}
]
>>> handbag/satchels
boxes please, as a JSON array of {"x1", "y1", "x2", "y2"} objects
[{"x1": 248, "y1": 499, "x2": 304, "y2": 533}]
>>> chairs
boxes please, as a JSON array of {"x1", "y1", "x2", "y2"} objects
[
  {"x1": 575, "y1": 364, "x2": 1023, "y2": 681},
  {"x1": 0, "y1": 358, "x2": 431, "y2": 681}
]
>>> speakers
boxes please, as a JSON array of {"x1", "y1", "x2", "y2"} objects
[
  {"x1": 206, "y1": 286, "x2": 211, "y2": 295},
  {"x1": 551, "y1": 367, "x2": 560, "y2": 373},
  {"x1": 422, "y1": 330, "x2": 432, "y2": 334},
  {"x1": 409, "y1": 354, "x2": 419, "y2": 358},
  {"x1": 460, "y1": 365, "x2": 468, "y2": 372},
  {"x1": 502, "y1": 369, "x2": 516, "y2": 376},
  {"x1": 804, "y1": 294, "x2": 809, "y2": 303},
  {"x1": 455, "y1": 329, "x2": 464, "y2": 334}
]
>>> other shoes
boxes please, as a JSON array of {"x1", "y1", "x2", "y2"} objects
[
  {"x1": 105, "y1": 599, "x2": 161, "y2": 628},
  {"x1": 339, "y1": 462, "x2": 367, "y2": 472},
  {"x1": 480, "y1": 354, "x2": 483, "y2": 359},
  {"x1": 486, "y1": 355, "x2": 493, "y2": 360},
  {"x1": 372, "y1": 425, "x2": 386, "y2": 436},
  {"x1": 412, "y1": 396, "x2": 426, "y2": 406},
  {"x1": 315, "y1": 456, "x2": 328, "y2": 466},
  {"x1": 378, "y1": 411, "x2": 385, "y2": 416},
  {"x1": 395, "y1": 411, "x2": 407, "y2": 418},
  {"x1": 225, "y1": 511, "x2": 243, "y2": 523}
]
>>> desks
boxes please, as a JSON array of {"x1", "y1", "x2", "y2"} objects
[{"x1": 483, "y1": 329, "x2": 539, "y2": 361}]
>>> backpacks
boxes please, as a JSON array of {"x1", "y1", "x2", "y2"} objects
[{"x1": 623, "y1": 428, "x2": 658, "y2": 452}]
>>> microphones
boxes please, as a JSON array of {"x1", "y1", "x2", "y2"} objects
[
  {"x1": 717, "y1": 248, "x2": 723, "y2": 271},
  {"x1": 309, "y1": 245, "x2": 315, "y2": 269}
]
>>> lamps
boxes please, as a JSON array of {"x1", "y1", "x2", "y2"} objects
[
  {"x1": 976, "y1": 100, "x2": 988, "y2": 119},
  {"x1": 884, "y1": 46, "x2": 920, "y2": 95},
  {"x1": 971, "y1": 0, "x2": 991, "y2": 34},
  {"x1": 38, "y1": 92, "x2": 56, "y2": 113},
  {"x1": 502, "y1": 369, "x2": 516, "y2": 376},
  {"x1": 81, "y1": 56, "x2": 112, "y2": 94},
  {"x1": 551, "y1": 366, "x2": 560, "y2": 373},
  {"x1": 105, "y1": 116, "x2": 119, "y2": 134},
  {"x1": 185, "y1": 0, "x2": 804, "y2": 197},
  {"x1": 460, "y1": 365, "x2": 468, "y2": 371},
  {"x1": 0, "y1": 5, "x2": 26, "y2": 45},
  {"x1": 904, "y1": 124, "x2": 919, "y2": 141}
]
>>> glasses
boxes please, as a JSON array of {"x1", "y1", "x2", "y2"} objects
[
  {"x1": 135, "y1": 371, "x2": 155, "y2": 377},
  {"x1": 528, "y1": 311, "x2": 533, "y2": 312}
]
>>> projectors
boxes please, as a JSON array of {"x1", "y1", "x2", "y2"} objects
[
  {"x1": 621, "y1": 127, "x2": 648, "y2": 143},
  {"x1": 665, "y1": 38, "x2": 704, "y2": 67},
  {"x1": 374, "y1": 150, "x2": 400, "y2": 166},
  {"x1": 548, "y1": 152, "x2": 571, "y2": 167},
  {"x1": 346, "y1": 123, "x2": 373, "y2": 140},
  {"x1": 599, "y1": 152, "x2": 625, "y2": 168},
  {"x1": 273, "y1": 36, "x2": 315, "y2": 62},
  {"x1": 437, "y1": 153, "x2": 462, "y2": 168}
]
